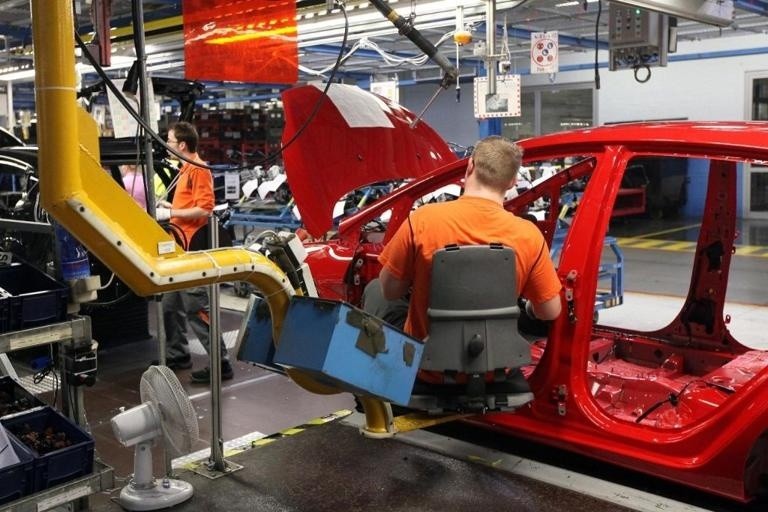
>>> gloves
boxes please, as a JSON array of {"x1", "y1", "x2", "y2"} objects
[{"x1": 155, "y1": 209, "x2": 171, "y2": 221}]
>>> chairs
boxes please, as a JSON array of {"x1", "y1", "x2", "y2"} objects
[{"x1": 404, "y1": 241, "x2": 534, "y2": 415}]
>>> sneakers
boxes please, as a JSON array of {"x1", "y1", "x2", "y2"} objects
[
  {"x1": 188, "y1": 359, "x2": 234, "y2": 382},
  {"x1": 152, "y1": 353, "x2": 192, "y2": 369}
]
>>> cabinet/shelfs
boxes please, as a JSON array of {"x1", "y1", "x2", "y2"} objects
[{"x1": 0, "y1": 128, "x2": 115, "y2": 512}]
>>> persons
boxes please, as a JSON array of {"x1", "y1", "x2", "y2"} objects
[
  {"x1": 149, "y1": 120, "x2": 234, "y2": 384},
  {"x1": 120, "y1": 162, "x2": 148, "y2": 212},
  {"x1": 357, "y1": 133, "x2": 565, "y2": 387}
]
